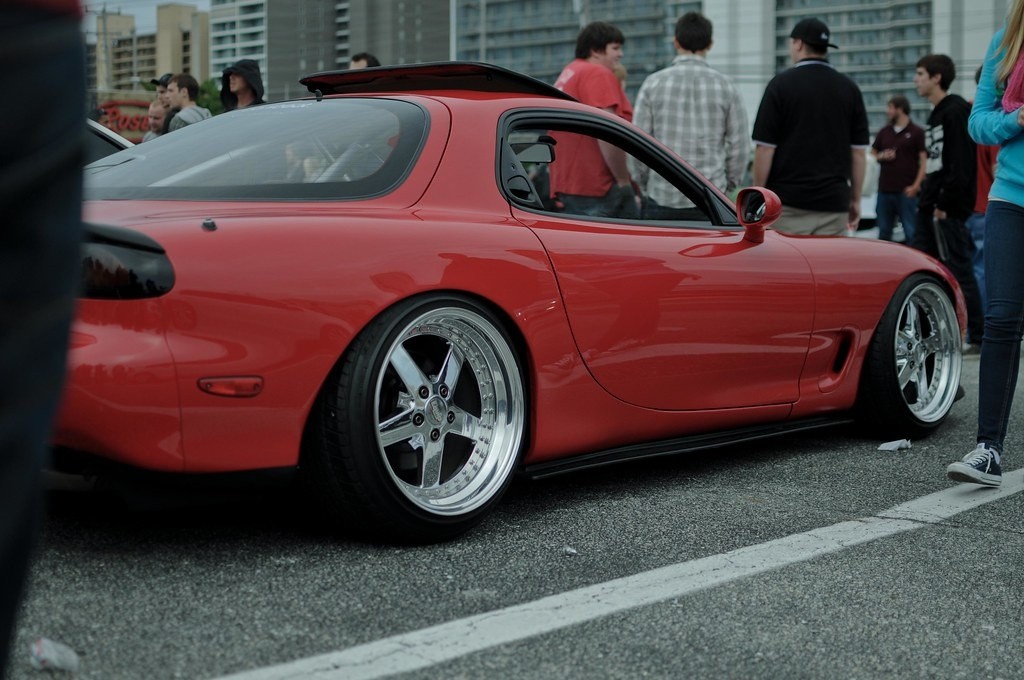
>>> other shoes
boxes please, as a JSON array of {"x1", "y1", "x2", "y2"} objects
[{"x1": 963, "y1": 342, "x2": 982, "y2": 356}]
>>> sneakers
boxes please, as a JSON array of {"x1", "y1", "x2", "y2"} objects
[{"x1": 947, "y1": 441, "x2": 1002, "y2": 486}]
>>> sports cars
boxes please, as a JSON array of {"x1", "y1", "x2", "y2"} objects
[{"x1": 43, "y1": 61, "x2": 970, "y2": 546}]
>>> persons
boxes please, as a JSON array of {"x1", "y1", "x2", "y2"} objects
[
  {"x1": 946, "y1": 0, "x2": 1024, "y2": 487},
  {"x1": 139, "y1": 48, "x2": 396, "y2": 195},
  {"x1": 0, "y1": 0, "x2": 87, "y2": 680},
  {"x1": 542, "y1": 15, "x2": 1006, "y2": 356}
]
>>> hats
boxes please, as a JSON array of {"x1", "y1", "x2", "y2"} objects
[
  {"x1": 790, "y1": 17, "x2": 839, "y2": 51},
  {"x1": 150, "y1": 73, "x2": 174, "y2": 87}
]
